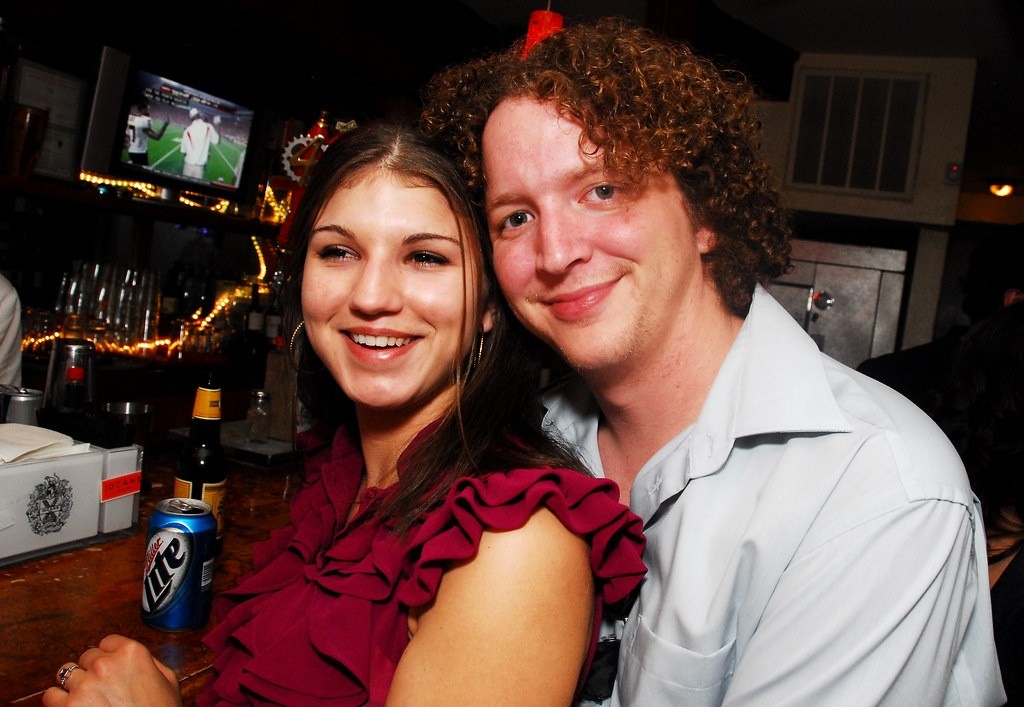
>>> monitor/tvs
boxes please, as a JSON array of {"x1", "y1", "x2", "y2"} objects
[{"x1": 70, "y1": 44, "x2": 271, "y2": 210}]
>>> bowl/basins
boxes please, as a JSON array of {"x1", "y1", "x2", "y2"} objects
[{"x1": 98, "y1": 400, "x2": 155, "y2": 431}]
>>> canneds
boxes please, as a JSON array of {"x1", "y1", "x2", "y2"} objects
[
  {"x1": 140, "y1": 497, "x2": 218, "y2": 631},
  {"x1": 0, "y1": 383, "x2": 44, "y2": 427}
]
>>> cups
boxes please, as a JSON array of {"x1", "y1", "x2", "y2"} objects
[{"x1": 42, "y1": 338, "x2": 97, "y2": 422}]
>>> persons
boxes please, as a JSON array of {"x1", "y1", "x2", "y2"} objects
[
  {"x1": 230, "y1": 129, "x2": 249, "y2": 187},
  {"x1": 0, "y1": 274, "x2": 21, "y2": 387},
  {"x1": 43, "y1": 121, "x2": 649, "y2": 707},
  {"x1": 419, "y1": 9, "x2": 1024, "y2": 707},
  {"x1": 126, "y1": 103, "x2": 171, "y2": 166},
  {"x1": 180, "y1": 108, "x2": 222, "y2": 181}
]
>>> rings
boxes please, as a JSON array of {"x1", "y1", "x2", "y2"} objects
[{"x1": 58, "y1": 665, "x2": 86, "y2": 693}]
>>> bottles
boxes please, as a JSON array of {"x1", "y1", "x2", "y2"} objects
[
  {"x1": 174, "y1": 371, "x2": 228, "y2": 559},
  {"x1": 269, "y1": 338, "x2": 286, "y2": 354},
  {"x1": 264, "y1": 291, "x2": 283, "y2": 336},
  {"x1": 244, "y1": 283, "x2": 264, "y2": 334},
  {"x1": 248, "y1": 389, "x2": 272, "y2": 444}
]
